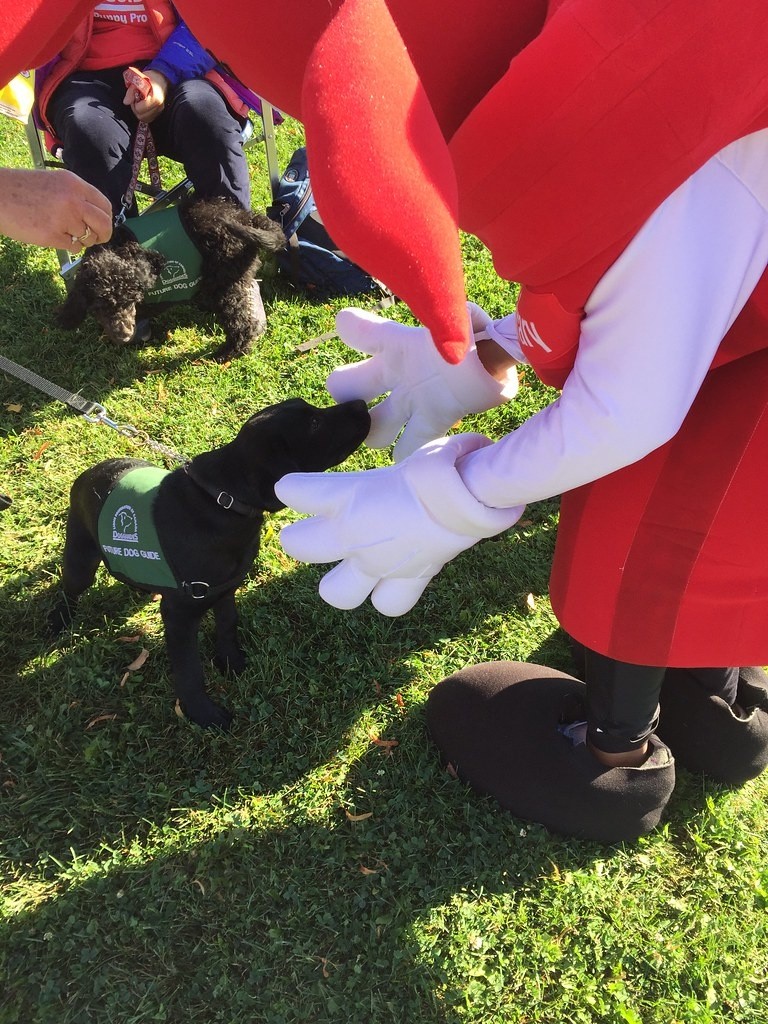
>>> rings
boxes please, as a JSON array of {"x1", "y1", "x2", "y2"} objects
[{"x1": 71, "y1": 225, "x2": 90, "y2": 246}]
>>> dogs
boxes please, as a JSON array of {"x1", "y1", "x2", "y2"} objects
[
  {"x1": 39, "y1": 397, "x2": 371, "y2": 735},
  {"x1": 51, "y1": 193, "x2": 287, "y2": 365}
]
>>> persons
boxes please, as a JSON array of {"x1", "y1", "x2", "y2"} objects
[
  {"x1": 0, "y1": 167, "x2": 114, "y2": 255},
  {"x1": 36, "y1": 0, "x2": 255, "y2": 343}
]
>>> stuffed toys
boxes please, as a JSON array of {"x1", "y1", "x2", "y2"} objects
[{"x1": 167, "y1": 3, "x2": 768, "y2": 1024}]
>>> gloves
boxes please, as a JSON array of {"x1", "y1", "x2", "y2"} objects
[
  {"x1": 273, "y1": 430, "x2": 530, "y2": 617},
  {"x1": 328, "y1": 302, "x2": 518, "y2": 464}
]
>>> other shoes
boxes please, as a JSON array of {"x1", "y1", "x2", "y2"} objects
[
  {"x1": 135, "y1": 304, "x2": 153, "y2": 342},
  {"x1": 571, "y1": 642, "x2": 767, "y2": 784},
  {"x1": 428, "y1": 659, "x2": 677, "y2": 842}
]
